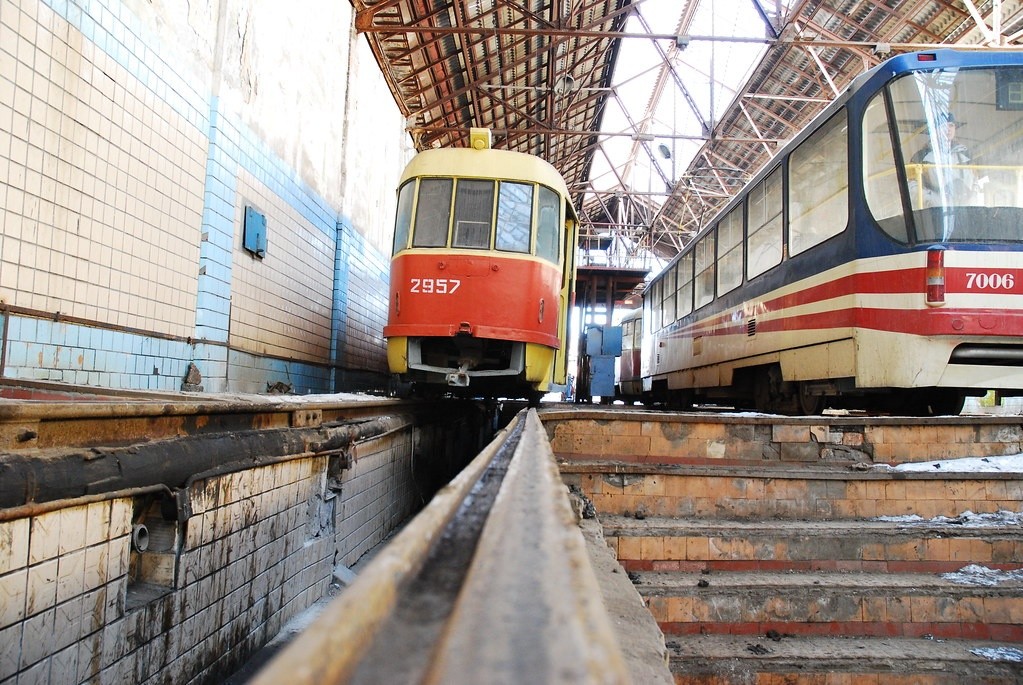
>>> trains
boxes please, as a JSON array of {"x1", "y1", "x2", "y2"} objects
[
  {"x1": 383, "y1": 127, "x2": 582, "y2": 403},
  {"x1": 617, "y1": 50, "x2": 1022, "y2": 414}
]
